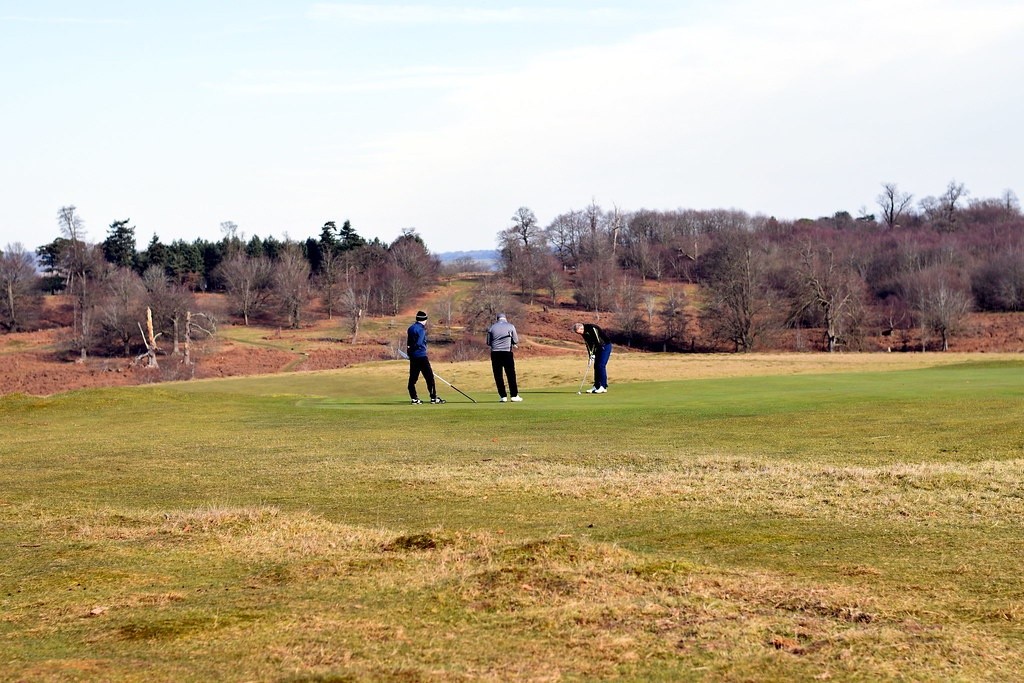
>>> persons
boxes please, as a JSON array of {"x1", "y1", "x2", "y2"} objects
[
  {"x1": 407, "y1": 311, "x2": 446, "y2": 404},
  {"x1": 574, "y1": 323, "x2": 612, "y2": 394},
  {"x1": 487, "y1": 314, "x2": 523, "y2": 402}
]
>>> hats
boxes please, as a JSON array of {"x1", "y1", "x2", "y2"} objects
[
  {"x1": 416, "y1": 311, "x2": 428, "y2": 321},
  {"x1": 497, "y1": 313, "x2": 506, "y2": 318}
]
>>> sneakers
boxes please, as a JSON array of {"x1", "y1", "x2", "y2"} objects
[
  {"x1": 431, "y1": 397, "x2": 446, "y2": 404},
  {"x1": 411, "y1": 399, "x2": 423, "y2": 405},
  {"x1": 586, "y1": 386, "x2": 607, "y2": 394},
  {"x1": 511, "y1": 395, "x2": 523, "y2": 402},
  {"x1": 499, "y1": 397, "x2": 507, "y2": 402}
]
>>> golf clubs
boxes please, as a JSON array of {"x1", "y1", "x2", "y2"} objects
[
  {"x1": 396, "y1": 348, "x2": 477, "y2": 403},
  {"x1": 576, "y1": 358, "x2": 592, "y2": 395}
]
